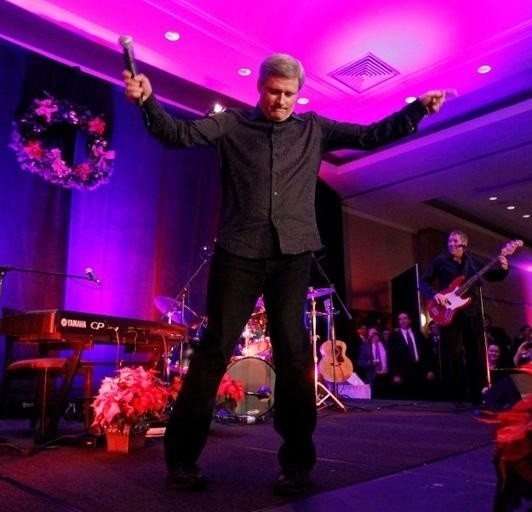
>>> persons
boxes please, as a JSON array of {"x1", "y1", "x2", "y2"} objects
[
  {"x1": 353, "y1": 310, "x2": 531, "y2": 400},
  {"x1": 416, "y1": 230, "x2": 510, "y2": 410},
  {"x1": 122, "y1": 54, "x2": 460, "y2": 496}
]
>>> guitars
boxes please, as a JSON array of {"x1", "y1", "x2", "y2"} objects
[
  {"x1": 319, "y1": 298, "x2": 353, "y2": 383},
  {"x1": 426, "y1": 240, "x2": 523, "y2": 326}
]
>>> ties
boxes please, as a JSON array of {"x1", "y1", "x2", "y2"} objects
[
  {"x1": 374, "y1": 344, "x2": 384, "y2": 372},
  {"x1": 406, "y1": 330, "x2": 417, "y2": 363}
]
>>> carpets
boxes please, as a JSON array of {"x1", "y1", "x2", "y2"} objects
[{"x1": 1, "y1": 398, "x2": 499, "y2": 512}]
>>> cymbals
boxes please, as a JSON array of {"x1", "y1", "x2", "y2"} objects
[
  {"x1": 308, "y1": 287, "x2": 335, "y2": 300},
  {"x1": 153, "y1": 296, "x2": 200, "y2": 327}
]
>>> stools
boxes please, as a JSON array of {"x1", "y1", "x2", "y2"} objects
[{"x1": 6, "y1": 358, "x2": 92, "y2": 436}]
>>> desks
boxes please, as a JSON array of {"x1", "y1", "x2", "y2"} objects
[{"x1": 1, "y1": 309, "x2": 191, "y2": 444}]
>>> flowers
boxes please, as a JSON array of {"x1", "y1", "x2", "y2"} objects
[
  {"x1": 5, "y1": 89, "x2": 116, "y2": 192},
  {"x1": 215, "y1": 373, "x2": 246, "y2": 413},
  {"x1": 87, "y1": 361, "x2": 185, "y2": 434}
]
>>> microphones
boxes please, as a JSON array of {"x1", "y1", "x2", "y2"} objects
[
  {"x1": 86, "y1": 267, "x2": 99, "y2": 285},
  {"x1": 118, "y1": 35, "x2": 143, "y2": 107}
]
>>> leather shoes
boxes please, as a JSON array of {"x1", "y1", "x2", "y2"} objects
[
  {"x1": 165, "y1": 462, "x2": 209, "y2": 491},
  {"x1": 274, "y1": 464, "x2": 313, "y2": 493}
]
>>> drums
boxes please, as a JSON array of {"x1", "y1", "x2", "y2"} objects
[
  {"x1": 236, "y1": 317, "x2": 269, "y2": 355},
  {"x1": 218, "y1": 355, "x2": 277, "y2": 418}
]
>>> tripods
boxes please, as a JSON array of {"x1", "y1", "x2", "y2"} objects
[{"x1": 310, "y1": 300, "x2": 372, "y2": 413}]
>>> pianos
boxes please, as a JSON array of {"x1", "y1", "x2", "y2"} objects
[{"x1": 0, "y1": 309, "x2": 185, "y2": 351}]
